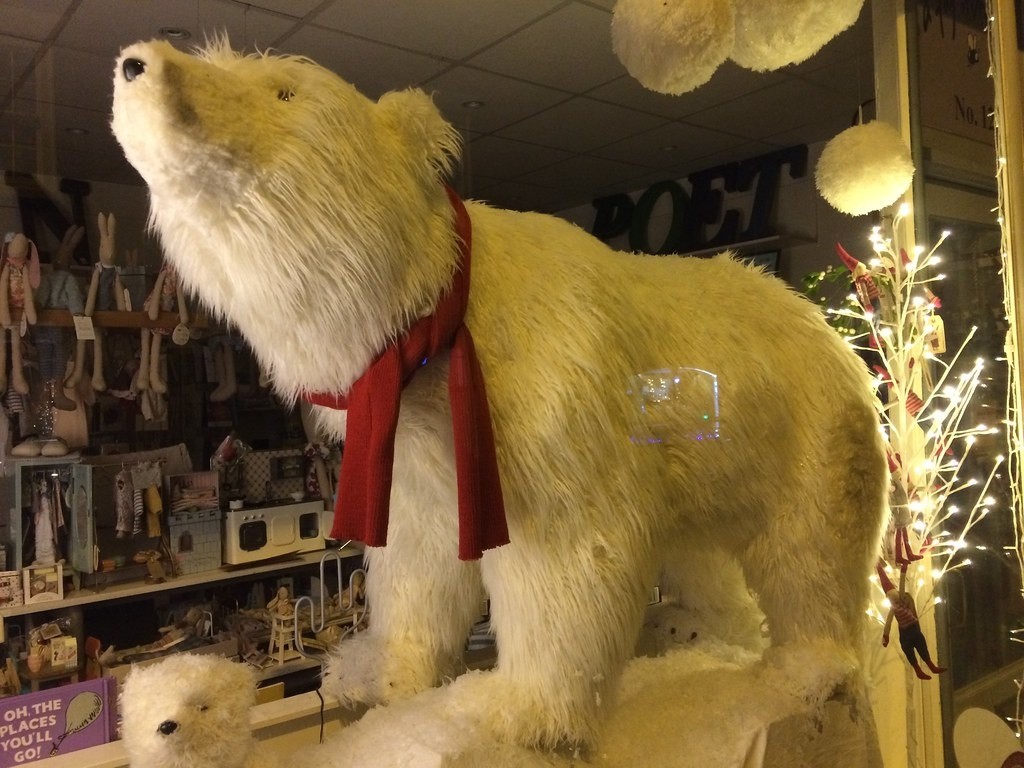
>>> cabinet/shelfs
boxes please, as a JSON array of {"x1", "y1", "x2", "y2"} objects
[{"x1": 0, "y1": 312, "x2": 363, "y2": 702}]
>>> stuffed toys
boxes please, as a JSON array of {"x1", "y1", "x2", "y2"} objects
[
  {"x1": 329, "y1": 570, "x2": 365, "y2": 618},
  {"x1": 114, "y1": 649, "x2": 280, "y2": 768},
  {"x1": 104, "y1": 29, "x2": 893, "y2": 761},
  {"x1": 136, "y1": 603, "x2": 211, "y2": 654},
  {"x1": 0, "y1": 212, "x2": 308, "y2": 424},
  {"x1": 267, "y1": 587, "x2": 295, "y2": 628},
  {"x1": 836, "y1": 237, "x2": 955, "y2": 680}
]
